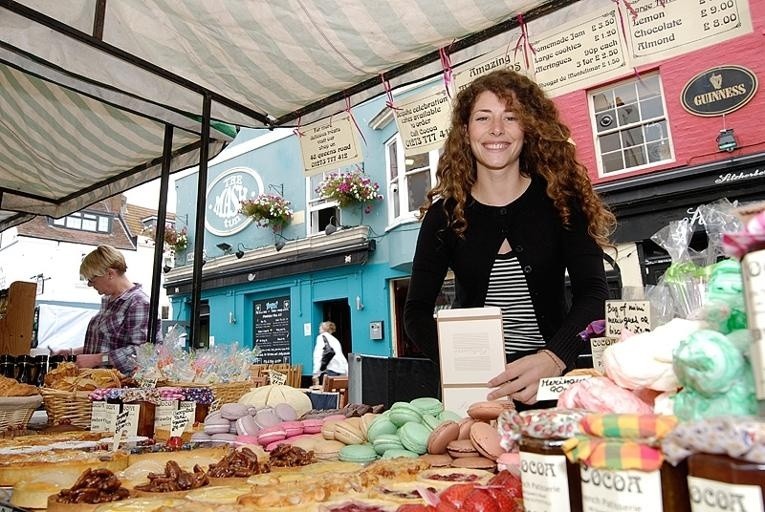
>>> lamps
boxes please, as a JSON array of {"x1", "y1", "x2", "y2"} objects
[
  {"x1": 715, "y1": 128, "x2": 737, "y2": 153},
  {"x1": 356, "y1": 296, "x2": 364, "y2": 310},
  {"x1": 235, "y1": 242, "x2": 253, "y2": 259},
  {"x1": 216, "y1": 242, "x2": 233, "y2": 254},
  {"x1": 325, "y1": 216, "x2": 340, "y2": 235},
  {"x1": 229, "y1": 311, "x2": 236, "y2": 324},
  {"x1": 274, "y1": 234, "x2": 294, "y2": 251},
  {"x1": 163, "y1": 263, "x2": 172, "y2": 274}
]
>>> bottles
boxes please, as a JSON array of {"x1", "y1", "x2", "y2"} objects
[{"x1": 1, "y1": 353, "x2": 77, "y2": 411}]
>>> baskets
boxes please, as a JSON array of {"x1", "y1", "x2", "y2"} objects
[{"x1": 0, "y1": 374, "x2": 257, "y2": 433}]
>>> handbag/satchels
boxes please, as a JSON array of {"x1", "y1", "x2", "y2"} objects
[{"x1": 321, "y1": 344, "x2": 335, "y2": 372}]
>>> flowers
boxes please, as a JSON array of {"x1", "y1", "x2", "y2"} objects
[
  {"x1": 142, "y1": 222, "x2": 188, "y2": 251},
  {"x1": 236, "y1": 193, "x2": 294, "y2": 234},
  {"x1": 314, "y1": 171, "x2": 384, "y2": 215}
]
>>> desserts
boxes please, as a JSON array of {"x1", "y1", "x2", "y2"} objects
[
  {"x1": 39, "y1": 363, "x2": 128, "y2": 426},
  {"x1": 339, "y1": 397, "x2": 463, "y2": 462},
  {"x1": 189, "y1": 402, "x2": 298, "y2": 449},
  {"x1": 0, "y1": 362, "x2": 43, "y2": 430},
  {"x1": 1, "y1": 424, "x2": 521, "y2": 512},
  {"x1": 238, "y1": 414, "x2": 345, "y2": 453},
  {"x1": 297, "y1": 403, "x2": 384, "y2": 421},
  {"x1": 132, "y1": 366, "x2": 269, "y2": 415},
  {"x1": 419, "y1": 399, "x2": 524, "y2": 480},
  {"x1": 292, "y1": 411, "x2": 379, "y2": 462}
]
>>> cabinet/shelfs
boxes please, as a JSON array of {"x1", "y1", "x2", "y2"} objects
[{"x1": 0, "y1": 282, "x2": 37, "y2": 356}]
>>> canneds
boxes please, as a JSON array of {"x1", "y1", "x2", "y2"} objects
[{"x1": 518, "y1": 436, "x2": 765, "y2": 512}]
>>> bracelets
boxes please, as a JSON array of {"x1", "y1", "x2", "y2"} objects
[{"x1": 538, "y1": 350, "x2": 562, "y2": 376}]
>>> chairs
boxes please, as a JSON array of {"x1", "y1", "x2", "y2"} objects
[
  {"x1": 252, "y1": 376, "x2": 267, "y2": 387},
  {"x1": 321, "y1": 373, "x2": 348, "y2": 408}
]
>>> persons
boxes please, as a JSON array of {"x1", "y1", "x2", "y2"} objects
[
  {"x1": 312, "y1": 321, "x2": 348, "y2": 385},
  {"x1": 77, "y1": 243, "x2": 152, "y2": 374},
  {"x1": 404, "y1": 67, "x2": 612, "y2": 411}
]
